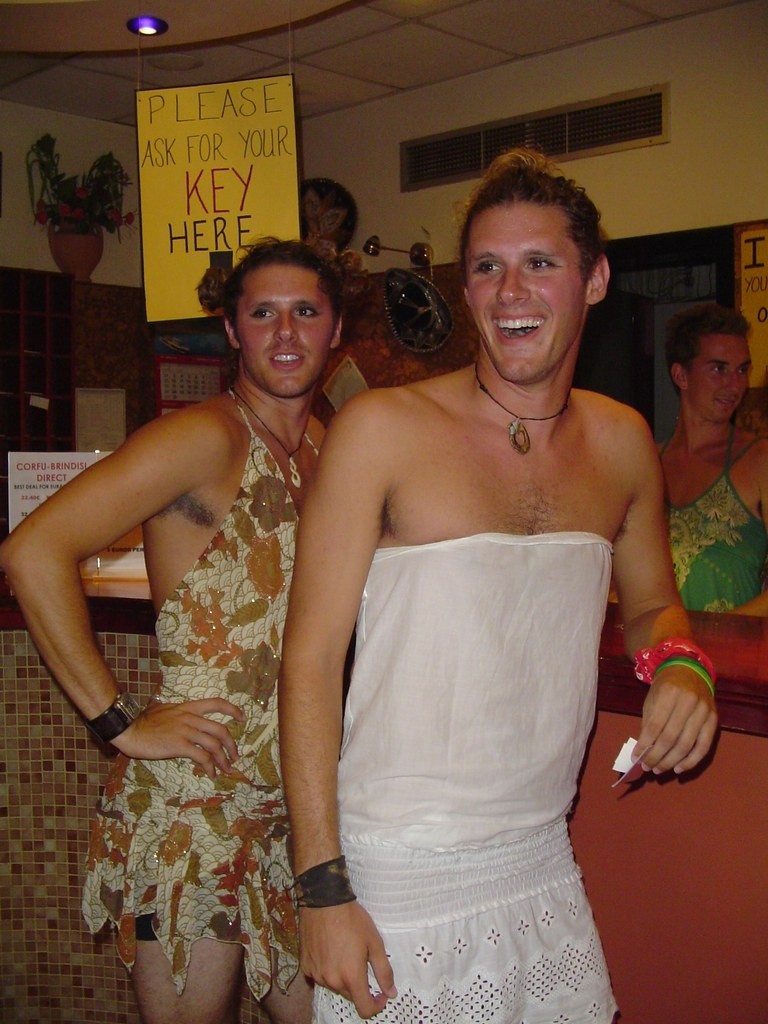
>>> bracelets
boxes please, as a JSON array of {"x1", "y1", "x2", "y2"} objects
[
  {"x1": 633, "y1": 637, "x2": 717, "y2": 696},
  {"x1": 294, "y1": 855, "x2": 358, "y2": 908}
]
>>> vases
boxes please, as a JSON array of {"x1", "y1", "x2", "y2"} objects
[{"x1": 51, "y1": 216, "x2": 105, "y2": 284}]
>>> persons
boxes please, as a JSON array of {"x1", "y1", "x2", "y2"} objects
[
  {"x1": 654, "y1": 305, "x2": 768, "y2": 615},
  {"x1": 277, "y1": 150, "x2": 718, "y2": 1024},
  {"x1": 0, "y1": 236, "x2": 343, "y2": 1024}
]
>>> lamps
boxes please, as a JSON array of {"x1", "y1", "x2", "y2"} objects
[{"x1": 363, "y1": 236, "x2": 435, "y2": 267}]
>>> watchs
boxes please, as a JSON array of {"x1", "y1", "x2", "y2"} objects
[{"x1": 85, "y1": 690, "x2": 141, "y2": 746}]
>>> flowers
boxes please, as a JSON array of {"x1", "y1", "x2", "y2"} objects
[{"x1": 21, "y1": 126, "x2": 135, "y2": 231}]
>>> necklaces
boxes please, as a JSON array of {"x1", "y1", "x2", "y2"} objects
[
  {"x1": 475, "y1": 360, "x2": 571, "y2": 455},
  {"x1": 230, "y1": 386, "x2": 309, "y2": 489}
]
